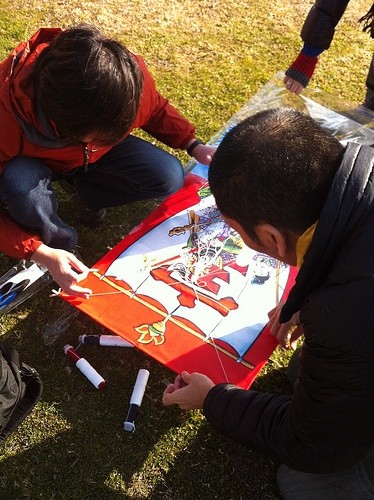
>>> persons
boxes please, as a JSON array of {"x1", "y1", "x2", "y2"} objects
[
  {"x1": 163, "y1": 108, "x2": 374, "y2": 500},
  {"x1": 1, "y1": 24, "x2": 222, "y2": 300},
  {"x1": 284, "y1": 0, "x2": 374, "y2": 124}
]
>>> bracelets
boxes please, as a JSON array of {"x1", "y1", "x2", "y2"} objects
[{"x1": 187, "y1": 139, "x2": 204, "y2": 158}]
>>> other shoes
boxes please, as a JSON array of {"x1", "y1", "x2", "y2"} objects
[{"x1": 351, "y1": 103, "x2": 374, "y2": 125}]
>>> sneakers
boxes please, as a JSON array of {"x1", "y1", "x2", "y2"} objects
[{"x1": 58, "y1": 181, "x2": 104, "y2": 226}]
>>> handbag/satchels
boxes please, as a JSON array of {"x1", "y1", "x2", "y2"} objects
[{"x1": 0, "y1": 343, "x2": 44, "y2": 444}]
